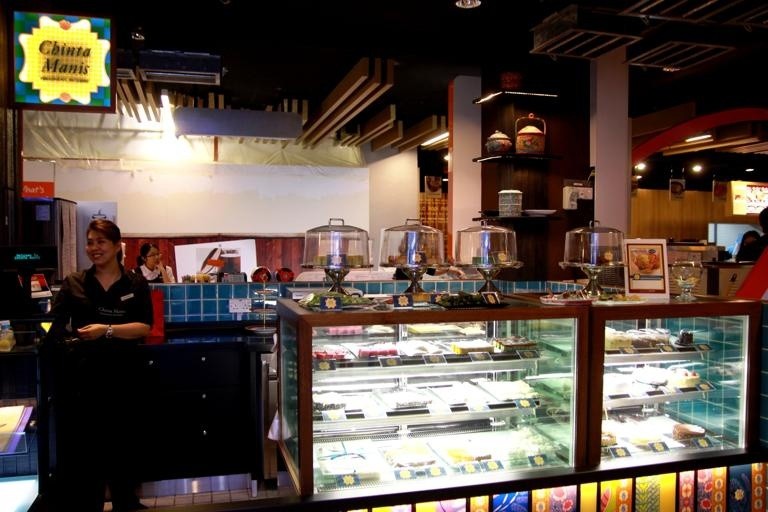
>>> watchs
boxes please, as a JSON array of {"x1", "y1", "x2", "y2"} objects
[{"x1": 105, "y1": 324, "x2": 114, "y2": 339}]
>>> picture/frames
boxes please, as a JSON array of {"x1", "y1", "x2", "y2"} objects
[{"x1": 623, "y1": 238, "x2": 670, "y2": 299}]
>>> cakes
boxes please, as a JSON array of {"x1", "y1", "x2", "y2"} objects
[
  {"x1": 312, "y1": 321, "x2": 710, "y2": 485},
  {"x1": 314, "y1": 245, "x2": 625, "y2": 267}
]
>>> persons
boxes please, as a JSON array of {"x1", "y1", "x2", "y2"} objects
[
  {"x1": 132, "y1": 242, "x2": 176, "y2": 284},
  {"x1": 39, "y1": 218, "x2": 156, "y2": 512},
  {"x1": 737, "y1": 207, "x2": 768, "y2": 261},
  {"x1": 741, "y1": 231, "x2": 761, "y2": 248}
]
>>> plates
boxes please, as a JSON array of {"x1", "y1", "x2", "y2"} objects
[
  {"x1": 424, "y1": 301, "x2": 511, "y2": 311},
  {"x1": 592, "y1": 296, "x2": 648, "y2": 307},
  {"x1": 538, "y1": 293, "x2": 598, "y2": 306},
  {"x1": 481, "y1": 210, "x2": 500, "y2": 216},
  {"x1": 523, "y1": 209, "x2": 558, "y2": 216}
]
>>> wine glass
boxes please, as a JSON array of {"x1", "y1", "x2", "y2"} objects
[{"x1": 671, "y1": 259, "x2": 704, "y2": 303}]
